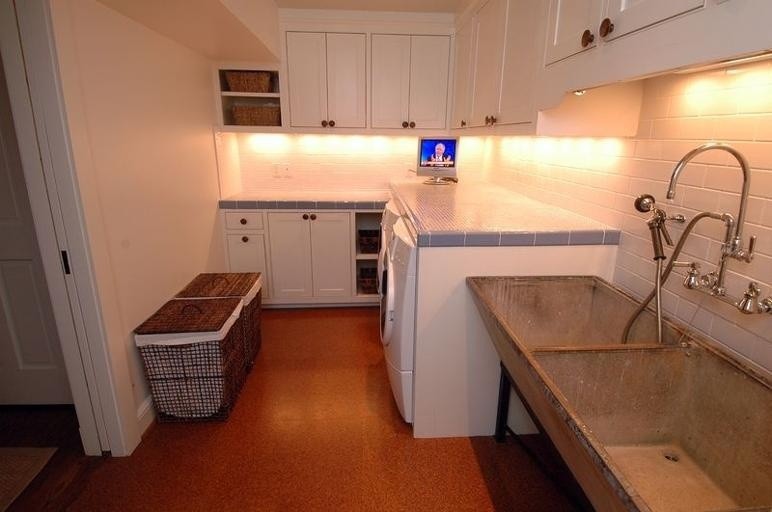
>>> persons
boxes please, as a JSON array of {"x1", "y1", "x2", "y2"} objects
[{"x1": 427, "y1": 143, "x2": 452, "y2": 167}]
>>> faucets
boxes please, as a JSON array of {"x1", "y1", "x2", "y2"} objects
[{"x1": 666, "y1": 143, "x2": 752, "y2": 251}]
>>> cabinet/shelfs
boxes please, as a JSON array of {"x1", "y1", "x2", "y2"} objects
[
  {"x1": 370, "y1": 31, "x2": 452, "y2": 133},
  {"x1": 217, "y1": 68, "x2": 283, "y2": 128},
  {"x1": 544, "y1": 0, "x2": 709, "y2": 94},
  {"x1": 286, "y1": 30, "x2": 369, "y2": 131},
  {"x1": 356, "y1": 207, "x2": 387, "y2": 307},
  {"x1": 266, "y1": 211, "x2": 353, "y2": 301},
  {"x1": 475, "y1": 1, "x2": 543, "y2": 126},
  {"x1": 452, "y1": 17, "x2": 474, "y2": 132},
  {"x1": 225, "y1": 211, "x2": 271, "y2": 301}
]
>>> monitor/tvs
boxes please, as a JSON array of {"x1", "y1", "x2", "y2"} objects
[{"x1": 417, "y1": 136, "x2": 459, "y2": 185}]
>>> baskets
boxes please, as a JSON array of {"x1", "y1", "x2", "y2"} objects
[
  {"x1": 225, "y1": 70, "x2": 273, "y2": 94},
  {"x1": 231, "y1": 103, "x2": 282, "y2": 127}
]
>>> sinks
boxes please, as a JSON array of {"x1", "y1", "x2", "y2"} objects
[
  {"x1": 531, "y1": 343, "x2": 768, "y2": 512},
  {"x1": 467, "y1": 276, "x2": 697, "y2": 347}
]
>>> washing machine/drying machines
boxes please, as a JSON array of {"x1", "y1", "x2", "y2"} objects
[
  {"x1": 376, "y1": 201, "x2": 402, "y2": 295},
  {"x1": 376, "y1": 201, "x2": 417, "y2": 426}
]
